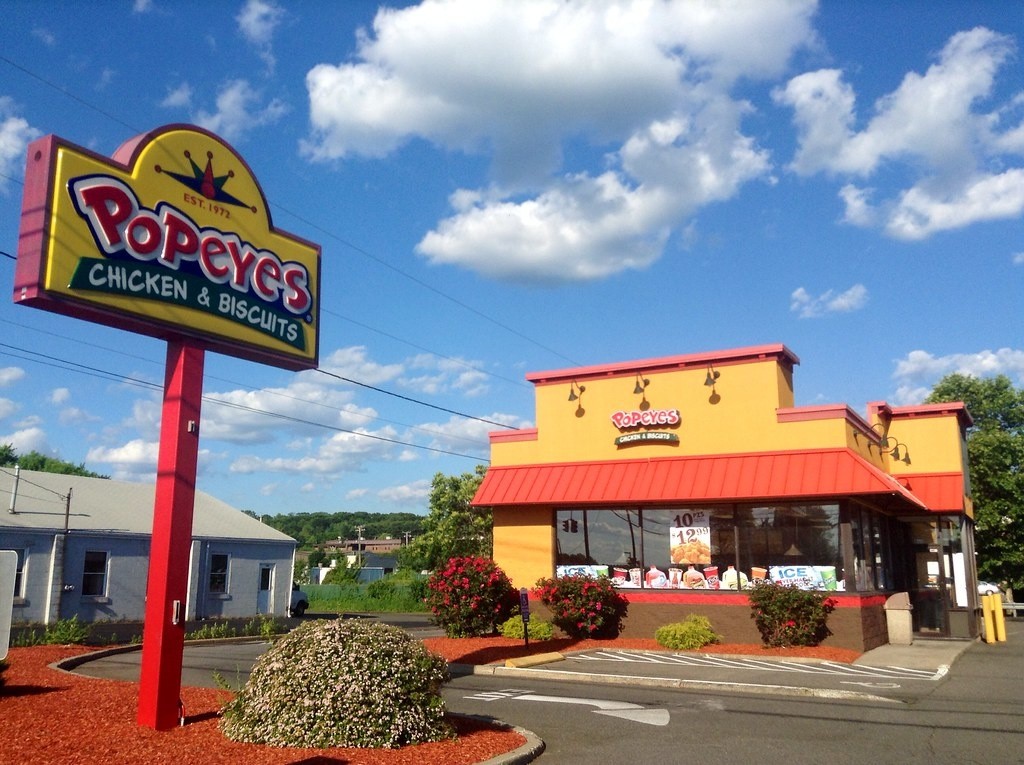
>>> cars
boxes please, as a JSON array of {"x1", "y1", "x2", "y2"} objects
[
  {"x1": 290, "y1": 581, "x2": 308, "y2": 615},
  {"x1": 978, "y1": 579, "x2": 999, "y2": 595}
]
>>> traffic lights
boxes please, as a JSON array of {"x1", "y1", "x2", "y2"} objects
[
  {"x1": 570, "y1": 520, "x2": 578, "y2": 533},
  {"x1": 563, "y1": 520, "x2": 568, "y2": 533}
]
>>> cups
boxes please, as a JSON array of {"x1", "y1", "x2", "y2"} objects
[
  {"x1": 613, "y1": 567, "x2": 628, "y2": 586},
  {"x1": 703, "y1": 567, "x2": 720, "y2": 590},
  {"x1": 751, "y1": 567, "x2": 767, "y2": 587},
  {"x1": 597, "y1": 566, "x2": 609, "y2": 580},
  {"x1": 821, "y1": 567, "x2": 837, "y2": 589},
  {"x1": 629, "y1": 568, "x2": 645, "y2": 588},
  {"x1": 668, "y1": 568, "x2": 683, "y2": 589}
]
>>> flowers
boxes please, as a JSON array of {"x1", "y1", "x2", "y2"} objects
[
  {"x1": 747, "y1": 578, "x2": 839, "y2": 649},
  {"x1": 531, "y1": 574, "x2": 631, "y2": 640},
  {"x1": 422, "y1": 554, "x2": 522, "y2": 639}
]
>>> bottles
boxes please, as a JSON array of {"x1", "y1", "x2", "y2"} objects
[
  {"x1": 722, "y1": 566, "x2": 748, "y2": 590},
  {"x1": 646, "y1": 565, "x2": 668, "y2": 589},
  {"x1": 683, "y1": 566, "x2": 706, "y2": 589}
]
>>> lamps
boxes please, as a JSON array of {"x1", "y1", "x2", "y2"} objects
[
  {"x1": 853, "y1": 423, "x2": 889, "y2": 450},
  {"x1": 704, "y1": 361, "x2": 720, "y2": 388},
  {"x1": 568, "y1": 379, "x2": 585, "y2": 402},
  {"x1": 634, "y1": 370, "x2": 650, "y2": 395},
  {"x1": 879, "y1": 443, "x2": 911, "y2": 466},
  {"x1": 868, "y1": 437, "x2": 900, "y2": 460}
]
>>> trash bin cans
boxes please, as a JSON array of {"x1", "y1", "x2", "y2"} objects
[{"x1": 883, "y1": 592, "x2": 913, "y2": 645}]
